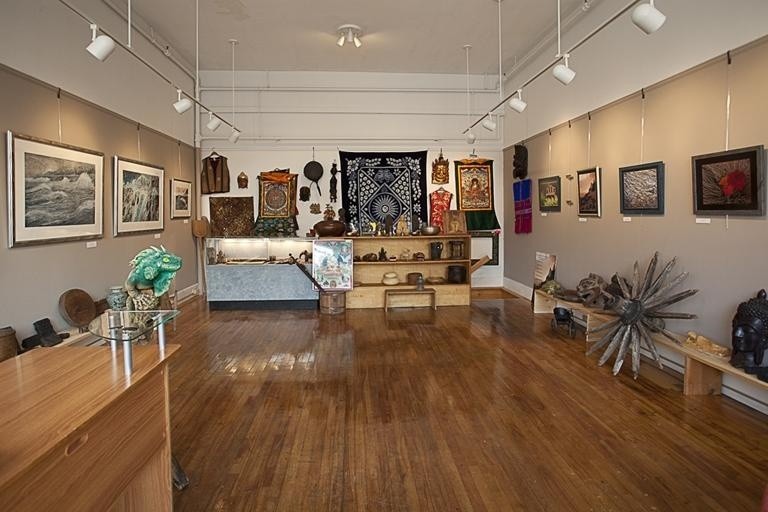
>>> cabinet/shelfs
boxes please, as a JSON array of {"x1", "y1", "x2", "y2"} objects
[
  {"x1": 319, "y1": 235, "x2": 471, "y2": 309},
  {"x1": 205, "y1": 237, "x2": 320, "y2": 311}
]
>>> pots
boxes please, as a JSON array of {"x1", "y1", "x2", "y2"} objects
[{"x1": 305, "y1": 160, "x2": 324, "y2": 199}]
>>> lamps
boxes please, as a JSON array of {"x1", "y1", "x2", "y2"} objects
[
  {"x1": 462, "y1": 0, "x2": 667, "y2": 144},
  {"x1": 336, "y1": 24, "x2": 362, "y2": 48},
  {"x1": 60, "y1": 0, "x2": 242, "y2": 145}
]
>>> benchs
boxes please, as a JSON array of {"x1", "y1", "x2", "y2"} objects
[
  {"x1": 384, "y1": 288, "x2": 436, "y2": 311},
  {"x1": 40, "y1": 327, "x2": 92, "y2": 347},
  {"x1": 655, "y1": 333, "x2": 768, "y2": 396},
  {"x1": 534, "y1": 289, "x2": 619, "y2": 342}
]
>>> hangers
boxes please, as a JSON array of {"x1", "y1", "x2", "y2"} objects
[
  {"x1": 437, "y1": 184, "x2": 446, "y2": 191},
  {"x1": 208, "y1": 147, "x2": 220, "y2": 157}
]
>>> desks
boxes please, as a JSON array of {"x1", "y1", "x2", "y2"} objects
[{"x1": 0, "y1": 310, "x2": 181, "y2": 512}]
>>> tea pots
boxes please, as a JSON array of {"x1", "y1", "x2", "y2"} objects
[{"x1": 431, "y1": 241, "x2": 444, "y2": 258}]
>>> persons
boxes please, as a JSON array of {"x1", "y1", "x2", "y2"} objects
[
  {"x1": 431, "y1": 147, "x2": 449, "y2": 185},
  {"x1": 728, "y1": 288, "x2": 768, "y2": 369}
]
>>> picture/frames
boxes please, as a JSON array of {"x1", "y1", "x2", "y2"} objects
[
  {"x1": 113, "y1": 155, "x2": 165, "y2": 237},
  {"x1": 538, "y1": 176, "x2": 561, "y2": 212},
  {"x1": 312, "y1": 240, "x2": 354, "y2": 291},
  {"x1": 577, "y1": 166, "x2": 600, "y2": 217},
  {"x1": 691, "y1": 144, "x2": 766, "y2": 216},
  {"x1": 171, "y1": 178, "x2": 192, "y2": 219},
  {"x1": 619, "y1": 161, "x2": 664, "y2": 215},
  {"x1": 468, "y1": 231, "x2": 499, "y2": 266},
  {"x1": 7, "y1": 129, "x2": 104, "y2": 248}
]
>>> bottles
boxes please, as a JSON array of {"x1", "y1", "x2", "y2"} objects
[{"x1": 416, "y1": 275, "x2": 424, "y2": 290}]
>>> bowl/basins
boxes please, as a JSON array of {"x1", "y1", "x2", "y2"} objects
[
  {"x1": 426, "y1": 276, "x2": 447, "y2": 285},
  {"x1": 421, "y1": 225, "x2": 441, "y2": 235}
]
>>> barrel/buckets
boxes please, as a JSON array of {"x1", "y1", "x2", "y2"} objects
[{"x1": 447, "y1": 265, "x2": 467, "y2": 284}]
